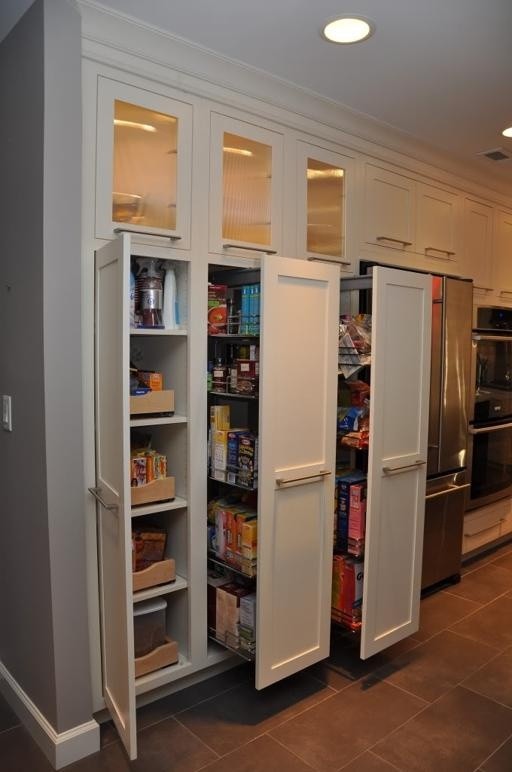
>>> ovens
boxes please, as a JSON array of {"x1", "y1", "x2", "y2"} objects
[{"x1": 464, "y1": 328, "x2": 511, "y2": 513}]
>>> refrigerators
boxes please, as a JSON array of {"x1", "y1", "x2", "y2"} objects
[{"x1": 423, "y1": 276, "x2": 477, "y2": 599}]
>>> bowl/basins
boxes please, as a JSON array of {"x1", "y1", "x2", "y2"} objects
[{"x1": 112, "y1": 191, "x2": 143, "y2": 223}]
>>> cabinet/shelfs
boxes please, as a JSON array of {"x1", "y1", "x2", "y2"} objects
[
  {"x1": 364, "y1": 163, "x2": 459, "y2": 265},
  {"x1": 91, "y1": 237, "x2": 433, "y2": 760},
  {"x1": 458, "y1": 194, "x2": 512, "y2": 308}
]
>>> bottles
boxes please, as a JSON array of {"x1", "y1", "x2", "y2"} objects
[{"x1": 213, "y1": 357, "x2": 229, "y2": 391}]
